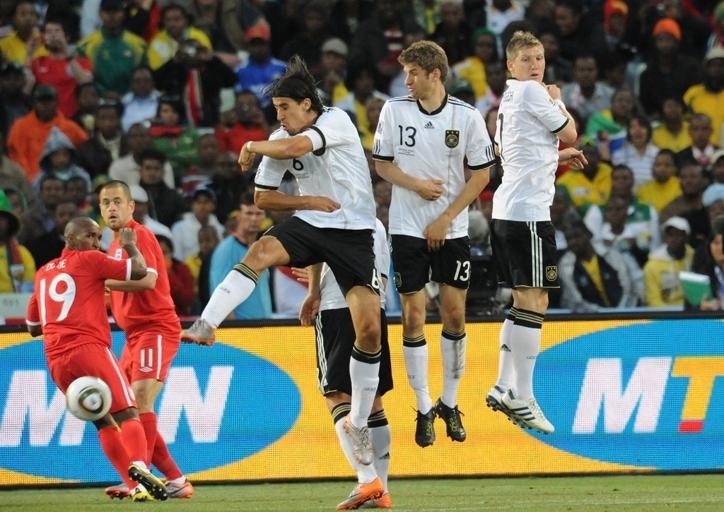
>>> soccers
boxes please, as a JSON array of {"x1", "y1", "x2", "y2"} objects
[{"x1": 66, "y1": 375, "x2": 112, "y2": 421}]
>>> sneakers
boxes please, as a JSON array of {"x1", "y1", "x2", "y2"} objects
[
  {"x1": 344, "y1": 414, "x2": 373, "y2": 465},
  {"x1": 336, "y1": 477, "x2": 391, "y2": 510},
  {"x1": 180, "y1": 319, "x2": 215, "y2": 345},
  {"x1": 105, "y1": 461, "x2": 194, "y2": 500},
  {"x1": 414, "y1": 389, "x2": 555, "y2": 446}
]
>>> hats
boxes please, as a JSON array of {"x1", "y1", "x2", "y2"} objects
[
  {"x1": 193, "y1": 186, "x2": 217, "y2": 201},
  {"x1": 130, "y1": 185, "x2": 148, "y2": 202},
  {"x1": 32, "y1": 87, "x2": 56, "y2": 101},
  {"x1": 702, "y1": 184, "x2": 724, "y2": 206},
  {"x1": 706, "y1": 49, "x2": 724, "y2": 61},
  {"x1": 663, "y1": 217, "x2": 691, "y2": 235},
  {"x1": 654, "y1": 20, "x2": 680, "y2": 39},
  {"x1": 322, "y1": 40, "x2": 348, "y2": 57},
  {"x1": 244, "y1": 28, "x2": 269, "y2": 44}
]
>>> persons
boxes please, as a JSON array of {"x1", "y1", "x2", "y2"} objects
[
  {"x1": 98, "y1": 180, "x2": 195, "y2": 500},
  {"x1": 26, "y1": 216, "x2": 169, "y2": 503},
  {"x1": 1, "y1": 2, "x2": 723, "y2": 325},
  {"x1": 484, "y1": 29, "x2": 589, "y2": 433},
  {"x1": 182, "y1": 53, "x2": 385, "y2": 466},
  {"x1": 370, "y1": 40, "x2": 498, "y2": 446},
  {"x1": 290, "y1": 216, "x2": 394, "y2": 510}
]
